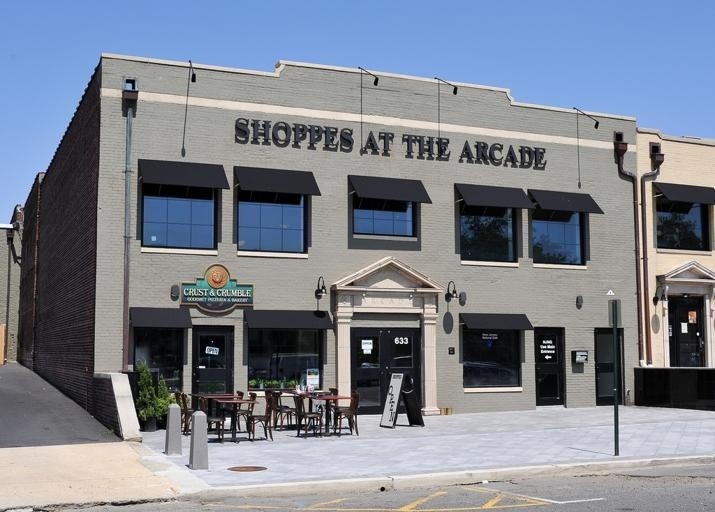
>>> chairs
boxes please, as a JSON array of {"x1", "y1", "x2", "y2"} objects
[
  {"x1": 175, "y1": 390, "x2": 274, "y2": 441},
  {"x1": 263, "y1": 385, "x2": 359, "y2": 436}
]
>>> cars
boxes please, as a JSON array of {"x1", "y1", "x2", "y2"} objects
[{"x1": 171, "y1": 355, "x2": 254, "y2": 386}]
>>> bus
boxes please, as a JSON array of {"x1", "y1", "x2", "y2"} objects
[{"x1": 267, "y1": 351, "x2": 319, "y2": 387}]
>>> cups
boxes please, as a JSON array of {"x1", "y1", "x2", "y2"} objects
[{"x1": 295, "y1": 384, "x2": 314, "y2": 396}]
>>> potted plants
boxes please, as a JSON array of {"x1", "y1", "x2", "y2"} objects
[{"x1": 134, "y1": 356, "x2": 177, "y2": 431}]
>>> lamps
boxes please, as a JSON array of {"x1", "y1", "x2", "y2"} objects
[
  {"x1": 315, "y1": 275, "x2": 327, "y2": 301},
  {"x1": 445, "y1": 280, "x2": 458, "y2": 303},
  {"x1": 653, "y1": 286, "x2": 668, "y2": 305}
]
